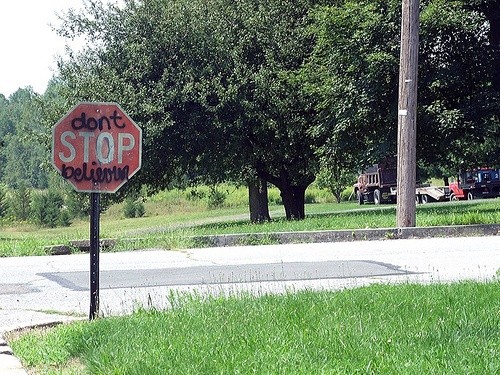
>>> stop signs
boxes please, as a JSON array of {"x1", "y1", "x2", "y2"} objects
[{"x1": 51, "y1": 102, "x2": 142, "y2": 193}]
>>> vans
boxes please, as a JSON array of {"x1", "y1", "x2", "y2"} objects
[{"x1": 428, "y1": 186, "x2": 449, "y2": 201}]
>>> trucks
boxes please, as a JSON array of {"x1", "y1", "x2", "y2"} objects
[
  {"x1": 353, "y1": 157, "x2": 445, "y2": 204},
  {"x1": 450, "y1": 161, "x2": 499, "y2": 201}
]
